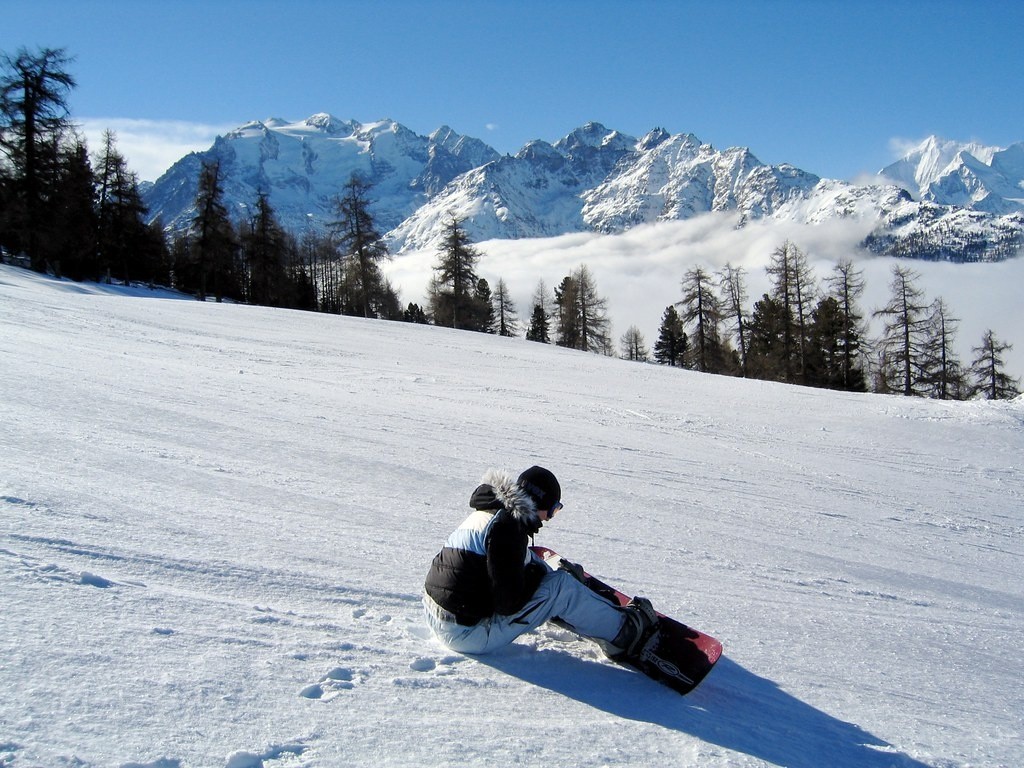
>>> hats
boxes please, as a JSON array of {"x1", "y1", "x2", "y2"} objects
[{"x1": 517, "y1": 466, "x2": 561, "y2": 511}]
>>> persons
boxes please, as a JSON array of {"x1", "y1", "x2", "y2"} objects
[{"x1": 421, "y1": 465, "x2": 656, "y2": 663}]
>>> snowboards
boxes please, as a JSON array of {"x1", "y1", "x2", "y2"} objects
[{"x1": 528, "y1": 545, "x2": 724, "y2": 697}]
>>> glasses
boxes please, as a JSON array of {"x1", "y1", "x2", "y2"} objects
[{"x1": 547, "y1": 503, "x2": 563, "y2": 518}]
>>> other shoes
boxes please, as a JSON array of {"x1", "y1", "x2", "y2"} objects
[{"x1": 605, "y1": 598, "x2": 653, "y2": 661}]
